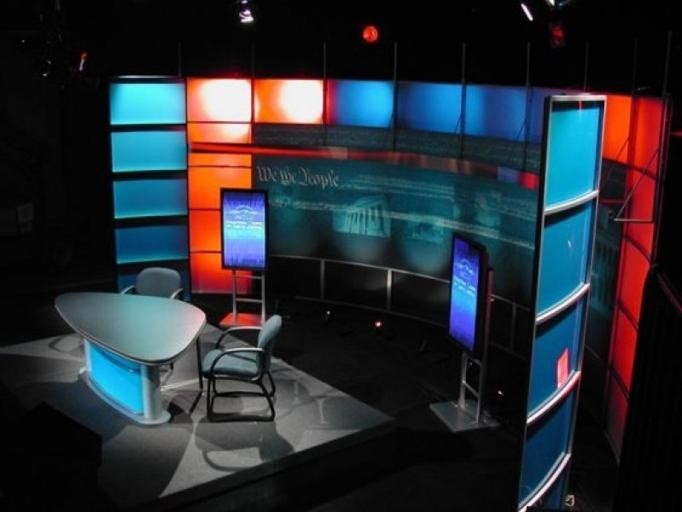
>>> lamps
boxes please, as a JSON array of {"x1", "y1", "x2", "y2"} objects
[
  {"x1": 238, "y1": 0, "x2": 255, "y2": 24},
  {"x1": 520, "y1": 0, "x2": 555, "y2": 22}
]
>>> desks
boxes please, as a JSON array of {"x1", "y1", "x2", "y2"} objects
[{"x1": 53, "y1": 291, "x2": 208, "y2": 426}]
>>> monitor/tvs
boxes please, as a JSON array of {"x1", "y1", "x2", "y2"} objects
[
  {"x1": 220, "y1": 187, "x2": 269, "y2": 272},
  {"x1": 444, "y1": 232, "x2": 488, "y2": 356}
]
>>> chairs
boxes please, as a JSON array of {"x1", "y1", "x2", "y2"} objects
[
  {"x1": 202, "y1": 314, "x2": 282, "y2": 422},
  {"x1": 121, "y1": 267, "x2": 184, "y2": 300}
]
